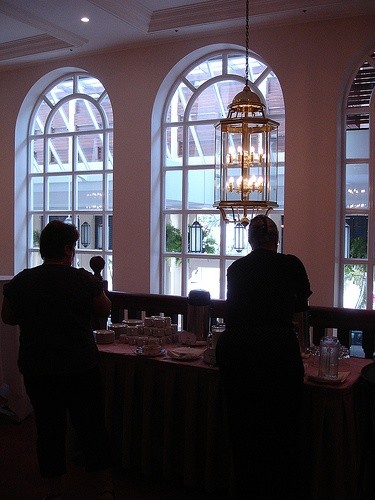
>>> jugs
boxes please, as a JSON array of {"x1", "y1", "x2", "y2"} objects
[
  {"x1": 318, "y1": 335, "x2": 340, "y2": 381},
  {"x1": 206, "y1": 324, "x2": 226, "y2": 350},
  {"x1": 183, "y1": 291, "x2": 217, "y2": 341}
]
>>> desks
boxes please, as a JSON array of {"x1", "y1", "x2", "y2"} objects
[{"x1": 93, "y1": 339, "x2": 375, "y2": 500}]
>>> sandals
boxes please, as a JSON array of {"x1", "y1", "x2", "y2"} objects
[
  {"x1": 98, "y1": 487, "x2": 115, "y2": 500},
  {"x1": 39, "y1": 492, "x2": 64, "y2": 500}
]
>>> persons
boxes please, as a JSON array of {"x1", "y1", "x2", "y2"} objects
[
  {"x1": 1, "y1": 220, "x2": 115, "y2": 500},
  {"x1": 215, "y1": 214, "x2": 309, "y2": 500}
]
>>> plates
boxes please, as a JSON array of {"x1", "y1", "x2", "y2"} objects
[
  {"x1": 136, "y1": 345, "x2": 165, "y2": 357},
  {"x1": 167, "y1": 347, "x2": 202, "y2": 360}
]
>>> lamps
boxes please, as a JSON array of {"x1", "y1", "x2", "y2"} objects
[
  {"x1": 188, "y1": 214, "x2": 203, "y2": 253},
  {"x1": 210, "y1": 0, "x2": 281, "y2": 230},
  {"x1": 80, "y1": 220, "x2": 92, "y2": 248}
]
